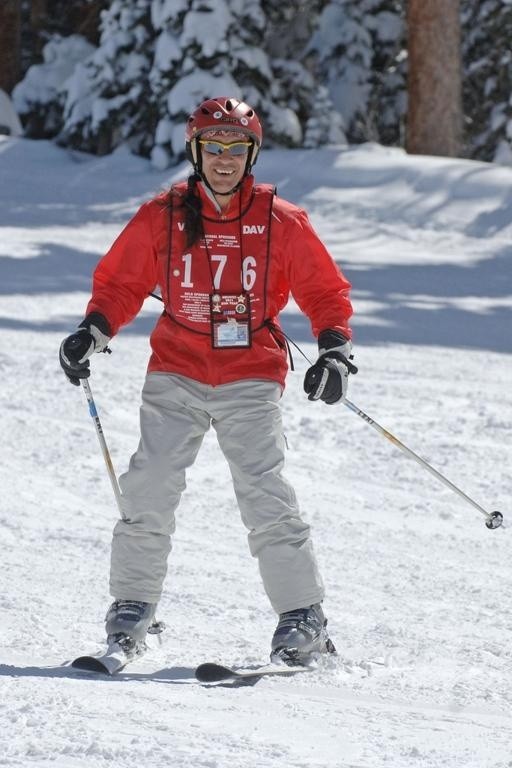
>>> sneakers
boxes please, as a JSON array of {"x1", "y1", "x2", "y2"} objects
[
  {"x1": 272, "y1": 603, "x2": 329, "y2": 654},
  {"x1": 105, "y1": 600, "x2": 156, "y2": 641}
]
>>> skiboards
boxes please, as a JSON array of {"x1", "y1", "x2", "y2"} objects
[{"x1": 71, "y1": 620, "x2": 344, "y2": 683}]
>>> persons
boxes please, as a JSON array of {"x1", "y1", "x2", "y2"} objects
[{"x1": 60, "y1": 99, "x2": 354, "y2": 665}]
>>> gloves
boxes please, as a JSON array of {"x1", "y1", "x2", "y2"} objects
[
  {"x1": 304, "y1": 329, "x2": 358, "y2": 405},
  {"x1": 60, "y1": 312, "x2": 112, "y2": 386}
]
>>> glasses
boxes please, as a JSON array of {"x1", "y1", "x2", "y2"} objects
[{"x1": 199, "y1": 141, "x2": 253, "y2": 156}]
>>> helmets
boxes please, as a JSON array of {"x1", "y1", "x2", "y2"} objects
[{"x1": 185, "y1": 97, "x2": 263, "y2": 177}]
